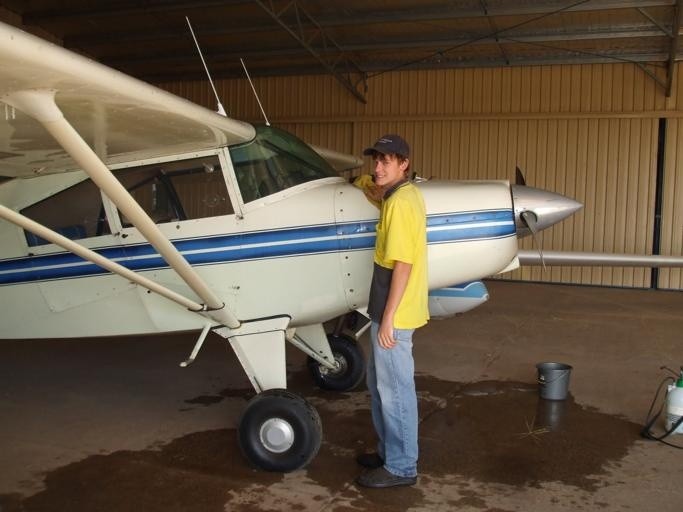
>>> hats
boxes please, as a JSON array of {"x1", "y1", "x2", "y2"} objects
[{"x1": 362, "y1": 134, "x2": 409, "y2": 159}]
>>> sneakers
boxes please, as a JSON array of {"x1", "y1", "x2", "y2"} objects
[
  {"x1": 356, "y1": 453, "x2": 383, "y2": 466},
  {"x1": 356, "y1": 467, "x2": 417, "y2": 489}
]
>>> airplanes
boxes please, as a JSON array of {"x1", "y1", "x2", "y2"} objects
[{"x1": 0, "y1": 0, "x2": 585, "y2": 479}]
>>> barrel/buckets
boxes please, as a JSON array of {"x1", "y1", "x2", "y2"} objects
[
  {"x1": 661, "y1": 365, "x2": 683, "y2": 435},
  {"x1": 536, "y1": 361, "x2": 574, "y2": 401}
]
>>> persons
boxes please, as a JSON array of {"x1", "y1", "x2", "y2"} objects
[{"x1": 355, "y1": 131, "x2": 432, "y2": 490}]
